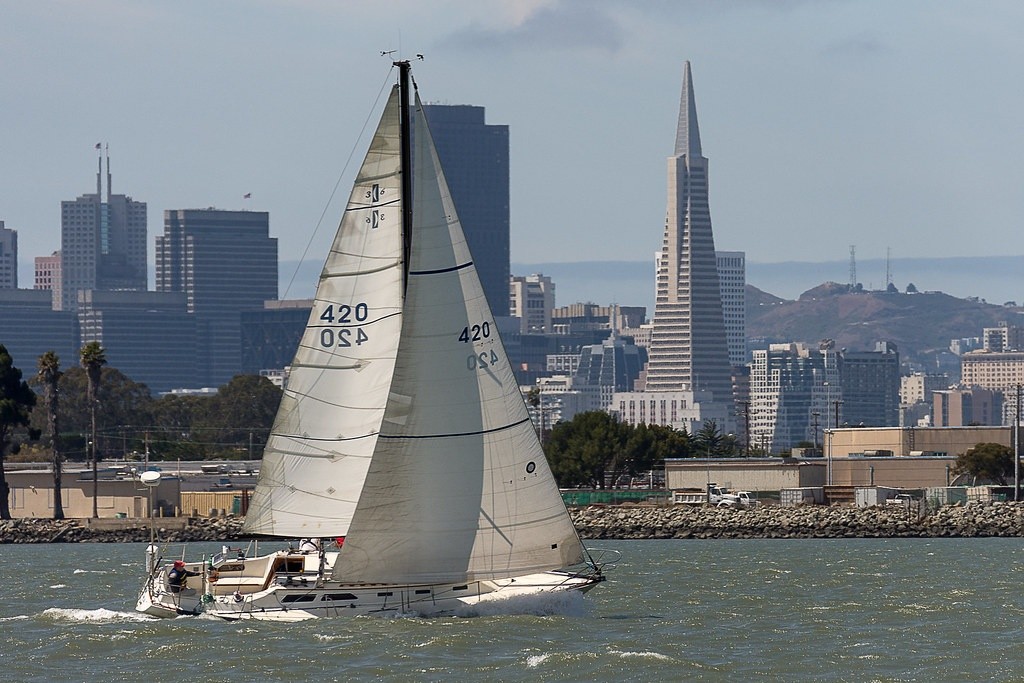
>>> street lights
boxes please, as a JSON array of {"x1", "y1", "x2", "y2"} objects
[{"x1": 824, "y1": 382, "x2": 830, "y2": 429}]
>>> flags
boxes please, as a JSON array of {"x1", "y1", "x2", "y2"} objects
[
  {"x1": 244, "y1": 193, "x2": 250, "y2": 199},
  {"x1": 95, "y1": 143, "x2": 109, "y2": 149}
]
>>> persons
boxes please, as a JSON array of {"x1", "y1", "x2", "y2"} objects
[{"x1": 168, "y1": 560, "x2": 204, "y2": 596}]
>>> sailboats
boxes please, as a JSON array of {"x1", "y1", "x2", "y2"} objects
[{"x1": 133, "y1": 58, "x2": 607, "y2": 622}]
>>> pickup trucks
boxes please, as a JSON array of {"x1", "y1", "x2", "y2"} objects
[{"x1": 885, "y1": 493, "x2": 921, "y2": 505}]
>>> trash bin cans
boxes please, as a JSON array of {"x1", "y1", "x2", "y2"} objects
[{"x1": 233, "y1": 496, "x2": 243, "y2": 512}]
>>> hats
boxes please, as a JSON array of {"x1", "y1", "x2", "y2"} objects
[{"x1": 174, "y1": 560, "x2": 185, "y2": 567}]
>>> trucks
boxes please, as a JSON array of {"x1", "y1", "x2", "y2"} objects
[{"x1": 671, "y1": 484, "x2": 755, "y2": 510}]
